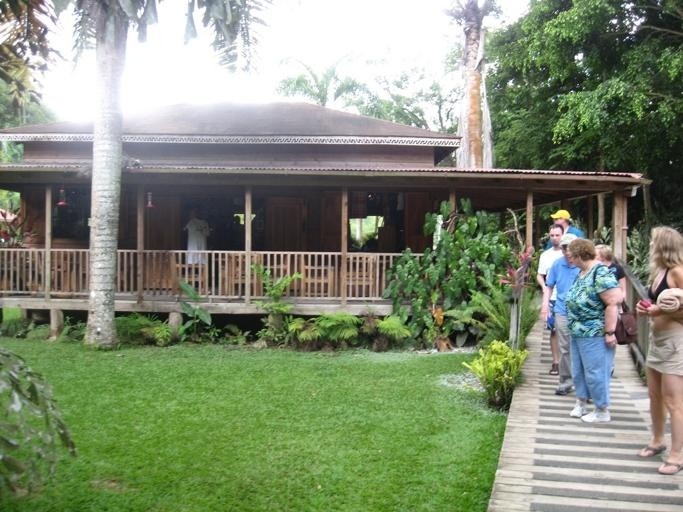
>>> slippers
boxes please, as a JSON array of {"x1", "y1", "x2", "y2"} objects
[
  {"x1": 639, "y1": 445, "x2": 666, "y2": 458},
  {"x1": 659, "y1": 462, "x2": 682, "y2": 474}
]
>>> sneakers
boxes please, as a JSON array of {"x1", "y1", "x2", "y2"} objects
[
  {"x1": 555, "y1": 384, "x2": 574, "y2": 394},
  {"x1": 570, "y1": 400, "x2": 586, "y2": 417},
  {"x1": 550, "y1": 362, "x2": 559, "y2": 375},
  {"x1": 581, "y1": 410, "x2": 610, "y2": 422}
]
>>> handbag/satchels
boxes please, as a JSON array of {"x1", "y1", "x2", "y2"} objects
[{"x1": 615, "y1": 314, "x2": 638, "y2": 345}]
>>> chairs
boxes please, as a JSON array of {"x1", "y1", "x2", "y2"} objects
[{"x1": 167, "y1": 252, "x2": 376, "y2": 300}]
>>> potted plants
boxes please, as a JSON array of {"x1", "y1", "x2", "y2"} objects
[
  {"x1": 247, "y1": 263, "x2": 303, "y2": 328},
  {"x1": 461, "y1": 338, "x2": 530, "y2": 411}
]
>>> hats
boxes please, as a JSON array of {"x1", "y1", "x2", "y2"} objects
[
  {"x1": 560, "y1": 234, "x2": 577, "y2": 246},
  {"x1": 551, "y1": 209, "x2": 571, "y2": 220}
]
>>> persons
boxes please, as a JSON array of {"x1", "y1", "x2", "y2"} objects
[
  {"x1": 186, "y1": 212, "x2": 210, "y2": 264},
  {"x1": 636, "y1": 226, "x2": 683, "y2": 475},
  {"x1": 537, "y1": 209, "x2": 626, "y2": 423}
]
